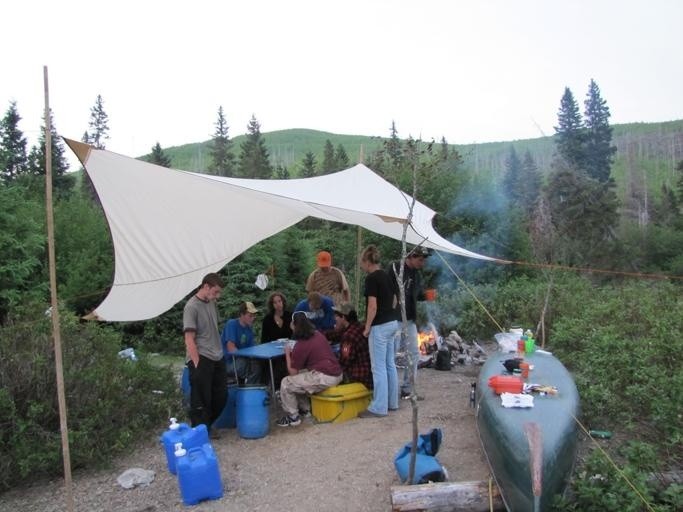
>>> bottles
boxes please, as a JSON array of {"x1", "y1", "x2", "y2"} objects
[
  {"x1": 512, "y1": 369, "x2": 521, "y2": 378},
  {"x1": 523, "y1": 330, "x2": 533, "y2": 340}
]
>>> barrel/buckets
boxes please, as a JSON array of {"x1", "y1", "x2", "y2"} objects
[
  {"x1": 235, "y1": 384, "x2": 271, "y2": 440},
  {"x1": 174, "y1": 442, "x2": 224, "y2": 506},
  {"x1": 181, "y1": 367, "x2": 193, "y2": 400},
  {"x1": 160, "y1": 417, "x2": 210, "y2": 475},
  {"x1": 488, "y1": 375, "x2": 523, "y2": 394},
  {"x1": 212, "y1": 382, "x2": 239, "y2": 429}
]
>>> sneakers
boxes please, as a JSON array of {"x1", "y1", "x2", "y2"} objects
[
  {"x1": 358, "y1": 409, "x2": 384, "y2": 417},
  {"x1": 276, "y1": 416, "x2": 301, "y2": 427}
]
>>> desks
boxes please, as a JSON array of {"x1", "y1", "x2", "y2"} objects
[{"x1": 226, "y1": 336, "x2": 345, "y2": 424}]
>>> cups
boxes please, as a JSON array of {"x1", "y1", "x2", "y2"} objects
[
  {"x1": 518, "y1": 340, "x2": 535, "y2": 353},
  {"x1": 425, "y1": 289, "x2": 435, "y2": 302},
  {"x1": 519, "y1": 364, "x2": 528, "y2": 378}
]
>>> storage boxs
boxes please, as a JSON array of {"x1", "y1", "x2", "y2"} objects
[{"x1": 311, "y1": 382, "x2": 372, "y2": 422}]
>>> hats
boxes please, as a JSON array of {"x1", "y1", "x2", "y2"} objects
[
  {"x1": 317, "y1": 252, "x2": 331, "y2": 268},
  {"x1": 331, "y1": 302, "x2": 355, "y2": 315},
  {"x1": 290, "y1": 311, "x2": 306, "y2": 330},
  {"x1": 413, "y1": 247, "x2": 432, "y2": 256},
  {"x1": 239, "y1": 302, "x2": 259, "y2": 314}
]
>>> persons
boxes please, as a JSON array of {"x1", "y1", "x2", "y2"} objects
[
  {"x1": 180, "y1": 274, "x2": 227, "y2": 432},
  {"x1": 357, "y1": 243, "x2": 398, "y2": 418},
  {"x1": 305, "y1": 251, "x2": 350, "y2": 306},
  {"x1": 220, "y1": 301, "x2": 263, "y2": 387},
  {"x1": 386, "y1": 245, "x2": 429, "y2": 401},
  {"x1": 289, "y1": 290, "x2": 335, "y2": 331},
  {"x1": 260, "y1": 291, "x2": 292, "y2": 343},
  {"x1": 319, "y1": 304, "x2": 372, "y2": 390},
  {"x1": 274, "y1": 311, "x2": 344, "y2": 427}
]
